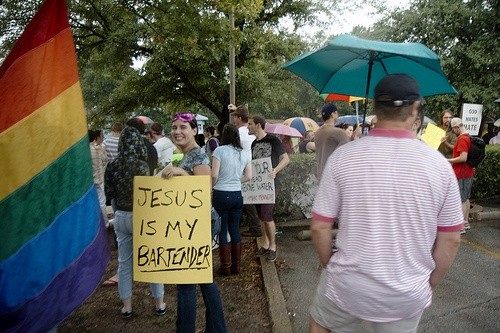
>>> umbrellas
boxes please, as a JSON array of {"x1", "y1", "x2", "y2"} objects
[
  {"x1": 422, "y1": 115, "x2": 438, "y2": 126},
  {"x1": 282, "y1": 35, "x2": 458, "y2": 137},
  {"x1": 265, "y1": 124, "x2": 302, "y2": 138},
  {"x1": 283, "y1": 117, "x2": 319, "y2": 134},
  {"x1": 334, "y1": 115, "x2": 363, "y2": 126},
  {"x1": 315, "y1": 92, "x2": 366, "y2": 126}
]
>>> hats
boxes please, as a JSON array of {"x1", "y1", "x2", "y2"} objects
[
  {"x1": 231, "y1": 105, "x2": 249, "y2": 117},
  {"x1": 375, "y1": 73, "x2": 419, "y2": 107},
  {"x1": 322, "y1": 104, "x2": 337, "y2": 120},
  {"x1": 446, "y1": 118, "x2": 462, "y2": 132}
]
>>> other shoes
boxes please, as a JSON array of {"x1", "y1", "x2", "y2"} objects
[
  {"x1": 242, "y1": 229, "x2": 262, "y2": 237},
  {"x1": 155, "y1": 302, "x2": 166, "y2": 315},
  {"x1": 460, "y1": 229, "x2": 466, "y2": 234},
  {"x1": 121, "y1": 307, "x2": 133, "y2": 318},
  {"x1": 267, "y1": 249, "x2": 277, "y2": 261},
  {"x1": 255, "y1": 246, "x2": 269, "y2": 257},
  {"x1": 464, "y1": 221, "x2": 471, "y2": 229}
]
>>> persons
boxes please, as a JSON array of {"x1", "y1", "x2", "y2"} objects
[{"x1": 88, "y1": 73, "x2": 500, "y2": 333}]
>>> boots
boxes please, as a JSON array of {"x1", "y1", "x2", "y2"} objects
[
  {"x1": 232, "y1": 244, "x2": 242, "y2": 274},
  {"x1": 217, "y1": 243, "x2": 232, "y2": 275}
]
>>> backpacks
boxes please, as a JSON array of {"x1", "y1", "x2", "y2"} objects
[{"x1": 456, "y1": 134, "x2": 486, "y2": 168}]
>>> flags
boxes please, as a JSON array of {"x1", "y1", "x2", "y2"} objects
[{"x1": 0, "y1": 0, "x2": 112, "y2": 333}]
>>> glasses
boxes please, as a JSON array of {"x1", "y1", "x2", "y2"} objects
[{"x1": 444, "y1": 117, "x2": 452, "y2": 118}]
